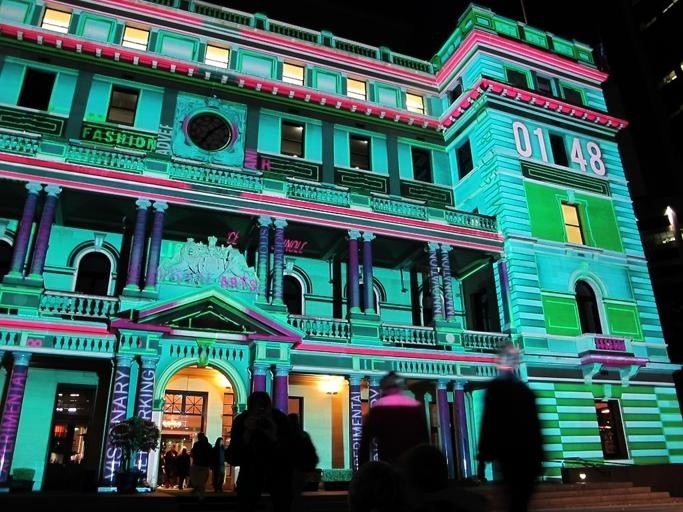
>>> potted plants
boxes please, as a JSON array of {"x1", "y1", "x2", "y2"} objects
[{"x1": 109, "y1": 416, "x2": 159, "y2": 494}]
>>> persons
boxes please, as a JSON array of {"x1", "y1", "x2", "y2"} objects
[
  {"x1": 393, "y1": 444, "x2": 487, "y2": 512},
  {"x1": 224, "y1": 389, "x2": 299, "y2": 511},
  {"x1": 357, "y1": 373, "x2": 431, "y2": 466},
  {"x1": 346, "y1": 458, "x2": 396, "y2": 511},
  {"x1": 157, "y1": 408, "x2": 320, "y2": 495},
  {"x1": 474, "y1": 342, "x2": 548, "y2": 512}
]
